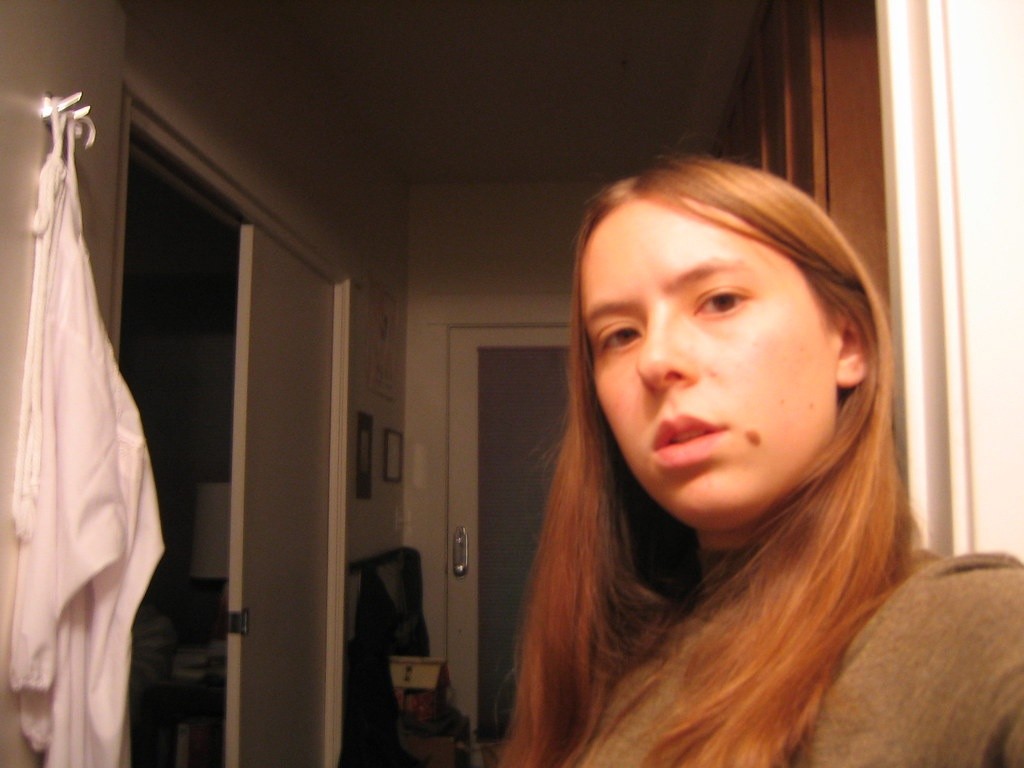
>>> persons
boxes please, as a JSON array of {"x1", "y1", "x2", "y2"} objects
[{"x1": 492, "y1": 158, "x2": 1024, "y2": 768}]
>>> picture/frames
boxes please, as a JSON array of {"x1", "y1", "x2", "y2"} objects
[
  {"x1": 384, "y1": 427, "x2": 403, "y2": 482},
  {"x1": 355, "y1": 410, "x2": 372, "y2": 500}
]
